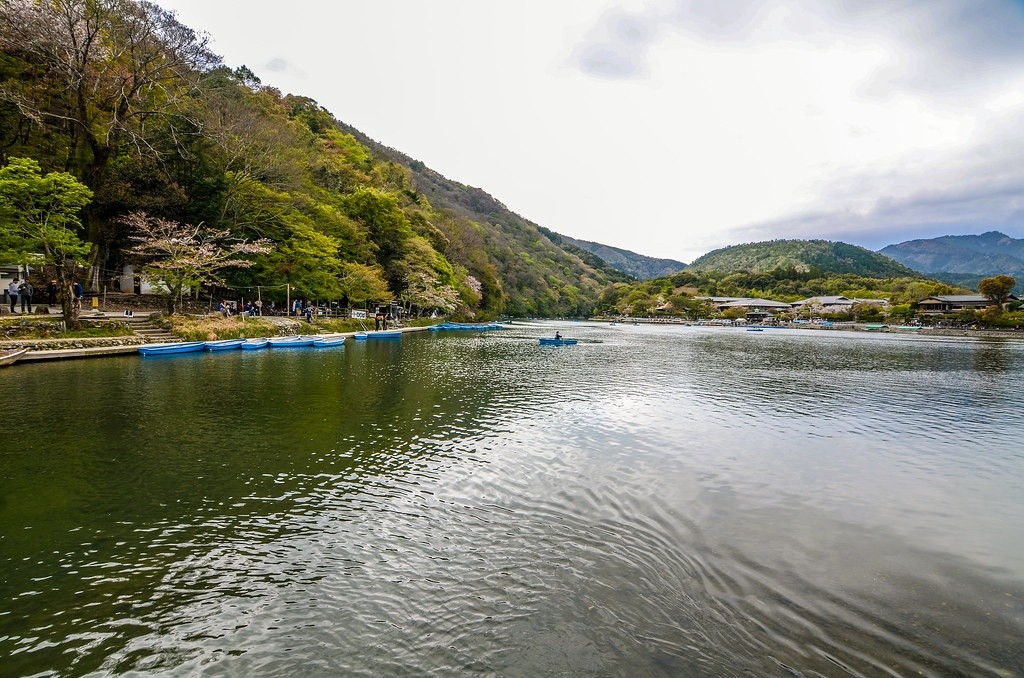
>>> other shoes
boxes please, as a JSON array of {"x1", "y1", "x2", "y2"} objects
[
  {"x1": 11, "y1": 311, "x2": 16, "y2": 313},
  {"x1": 50, "y1": 304, "x2": 56, "y2": 307}
]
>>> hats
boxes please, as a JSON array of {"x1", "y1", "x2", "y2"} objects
[{"x1": 13, "y1": 278, "x2": 19, "y2": 281}]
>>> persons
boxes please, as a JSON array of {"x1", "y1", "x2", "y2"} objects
[
  {"x1": 8, "y1": 278, "x2": 82, "y2": 313},
  {"x1": 375, "y1": 316, "x2": 387, "y2": 331},
  {"x1": 217, "y1": 296, "x2": 315, "y2": 323},
  {"x1": 555, "y1": 331, "x2": 562, "y2": 340}
]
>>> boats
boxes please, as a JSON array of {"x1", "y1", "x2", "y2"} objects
[
  {"x1": 539, "y1": 338, "x2": 577, "y2": 347},
  {"x1": 136, "y1": 309, "x2": 506, "y2": 356},
  {"x1": 747, "y1": 329, "x2": 763, "y2": 331}
]
